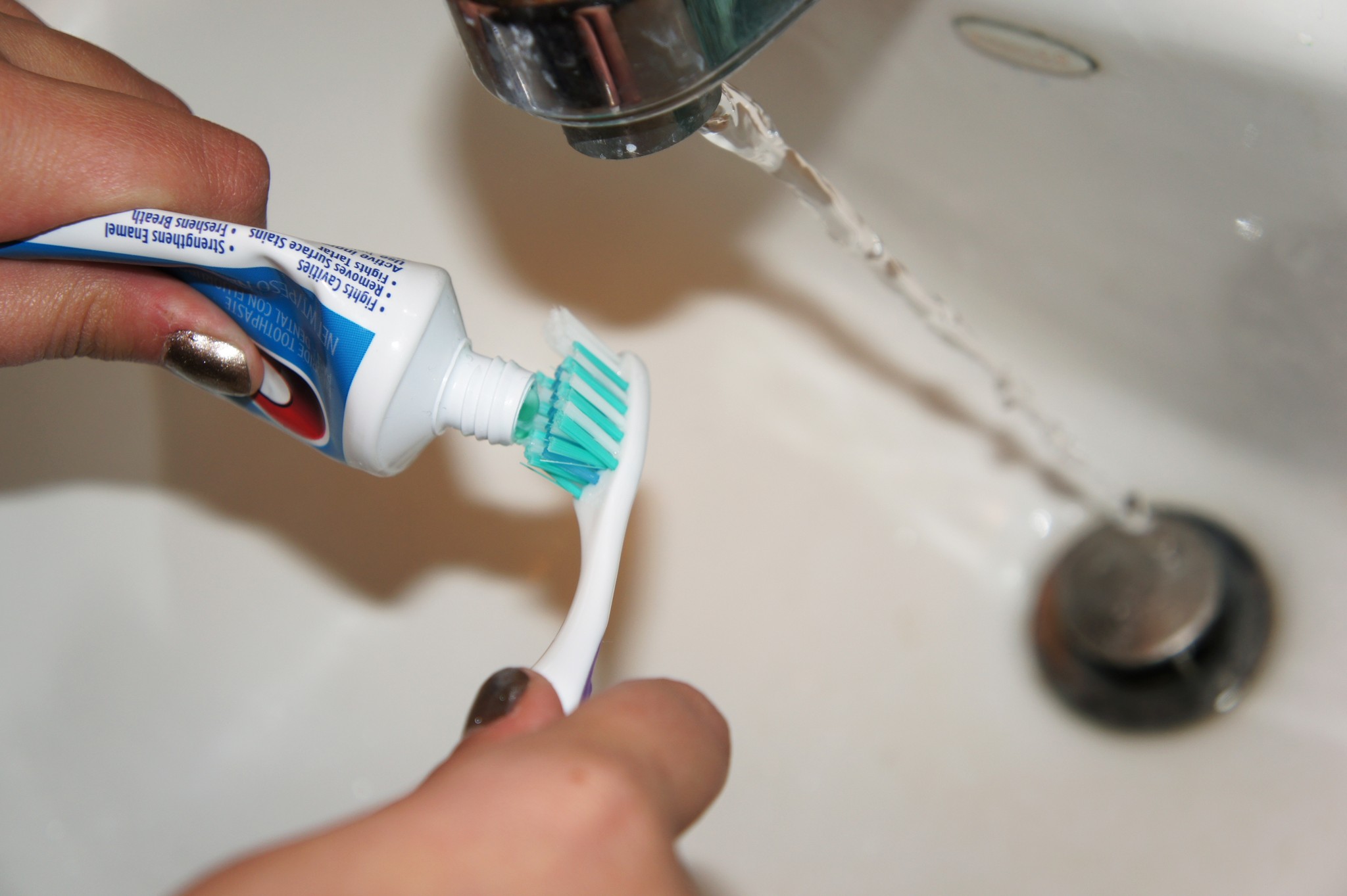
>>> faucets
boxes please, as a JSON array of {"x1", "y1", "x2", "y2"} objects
[{"x1": 442, "y1": 0, "x2": 817, "y2": 159}]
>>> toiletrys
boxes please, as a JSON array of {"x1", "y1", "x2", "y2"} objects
[{"x1": 3, "y1": 206, "x2": 542, "y2": 478}]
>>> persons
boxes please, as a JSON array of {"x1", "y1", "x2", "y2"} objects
[{"x1": 3, "y1": 3, "x2": 732, "y2": 896}]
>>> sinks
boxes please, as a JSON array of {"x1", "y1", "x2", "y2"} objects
[{"x1": 4, "y1": 0, "x2": 1347, "y2": 896}]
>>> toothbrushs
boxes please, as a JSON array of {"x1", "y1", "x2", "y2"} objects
[{"x1": 513, "y1": 302, "x2": 652, "y2": 729}]
